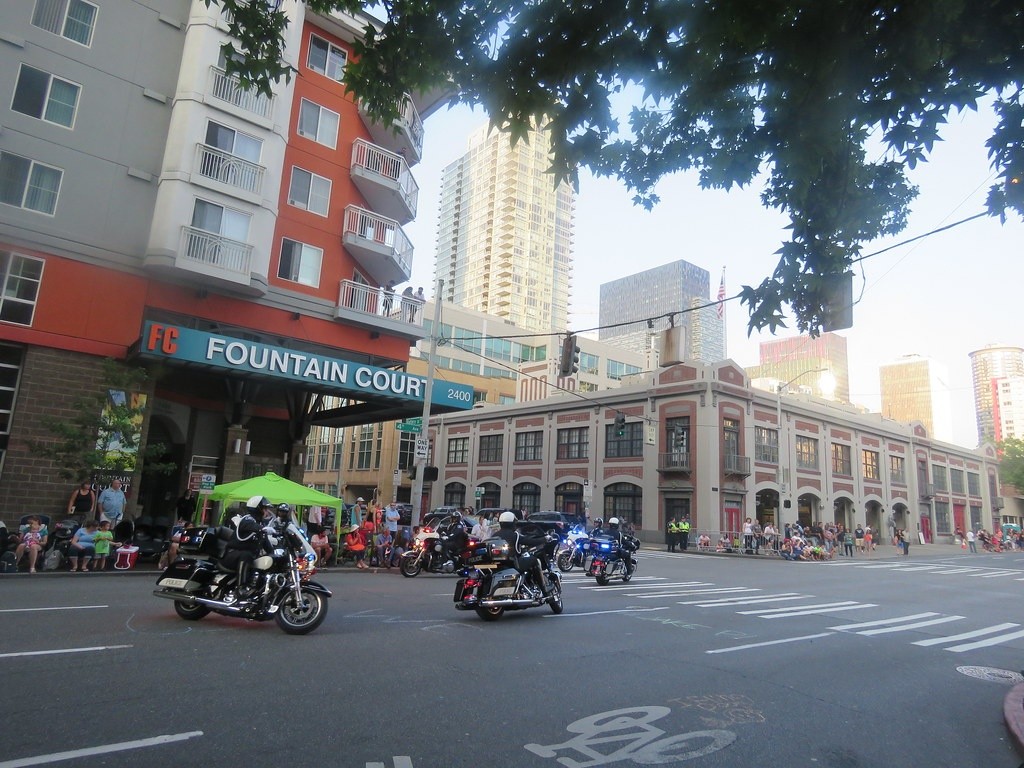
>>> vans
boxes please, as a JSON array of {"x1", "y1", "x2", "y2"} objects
[
  {"x1": 475, "y1": 508, "x2": 523, "y2": 521},
  {"x1": 524, "y1": 510, "x2": 578, "y2": 536}
]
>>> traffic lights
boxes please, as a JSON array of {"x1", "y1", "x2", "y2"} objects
[
  {"x1": 674, "y1": 425, "x2": 685, "y2": 451},
  {"x1": 560, "y1": 335, "x2": 580, "y2": 377},
  {"x1": 614, "y1": 411, "x2": 626, "y2": 437}
]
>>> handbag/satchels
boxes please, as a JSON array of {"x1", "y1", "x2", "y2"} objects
[{"x1": 46, "y1": 550, "x2": 61, "y2": 570}]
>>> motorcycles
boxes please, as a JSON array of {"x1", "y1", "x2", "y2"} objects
[
  {"x1": 399, "y1": 518, "x2": 478, "y2": 577},
  {"x1": 556, "y1": 522, "x2": 638, "y2": 586},
  {"x1": 152, "y1": 520, "x2": 333, "y2": 634},
  {"x1": 453, "y1": 524, "x2": 566, "y2": 620}
]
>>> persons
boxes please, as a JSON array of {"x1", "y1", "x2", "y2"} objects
[
  {"x1": 665, "y1": 513, "x2": 739, "y2": 553},
  {"x1": 0, "y1": 520, "x2": 9, "y2": 556},
  {"x1": 261, "y1": 502, "x2": 292, "y2": 536},
  {"x1": 393, "y1": 146, "x2": 408, "y2": 179},
  {"x1": 176, "y1": 488, "x2": 196, "y2": 522},
  {"x1": 492, "y1": 512, "x2": 552, "y2": 598},
  {"x1": 310, "y1": 526, "x2": 333, "y2": 567},
  {"x1": 586, "y1": 517, "x2": 604, "y2": 537},
  {"x1": 67, "y1": 479, "x2": 95, "y2": 524},
  {"x1": 346, "y1": 497, "x2": 420, "y2": 568},
  {"x1": 307, "y1": 506, "x2": 322, "y2": 533},
  {"x1": 781, "y1": 521, "x2": 878, "y2": 562},
  {"x1": 160, "y1": 522, "x2": 194, "y2": 569},
  {"x1": 606, "y1": 517, "x2": 635, "y2": 575},
  {"x1": 442, "y1": 510, "x2": 468, "y2": 570},
  {"x1": 98, "y1": 480, "x2": 127, "y2": 530},
  {"x1": 222, "y1": 495, "x2": 275, "y2": 599},
  {"x1": 742, "y1": 518, "x2": 782, "y2": 556},
  {"x1": 67, "y1": 519, "x2": 123, "y2": 571},
  {"x1": 956, "y1": 525, "x2": 1024, "y2": 553},
  {"x1": 382, "y1": 280, "x2": 426, "y2": 323},
  {"x1": 14, "y1": 514, "x2": 48, "y2": 573},
  {"x1": 895, "y1": 527, "x2": 911, "y2": 555},
  {"x1": 464, "y1": 506, "x2": 501, "y2": 542}
]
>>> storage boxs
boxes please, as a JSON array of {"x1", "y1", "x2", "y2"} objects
[{"x1": 114, "y1": 546, "x2": 140, "y2": 570}]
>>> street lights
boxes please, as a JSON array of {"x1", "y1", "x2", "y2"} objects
[{"x1": 778, "y1": 366, "x2": 829, "y2": 543}]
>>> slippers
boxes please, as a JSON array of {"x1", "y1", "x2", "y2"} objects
[
  {"x1": 70, "y1": 568, "x2": 77, "y2": 572},
  {"x1": 81, "y1": 567, "x2": 89, "y2": 571}
]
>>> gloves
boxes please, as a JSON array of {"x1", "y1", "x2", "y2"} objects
[
  {"x1": 442, "y1": 536, "x2": 449, "y2": 541},
  {"x1": 262, "y1": 527, "x2": 277, "y2": 536},
  {"x1": 544, "y1": 535, "x2": 553, "y2": 543}
]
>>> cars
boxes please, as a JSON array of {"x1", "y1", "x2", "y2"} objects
[
  {"x1": 423, "y1": 506, "x2": 458, "y2": 526},
  {"x1": 396, "y1": 504, "x2": 413, "y2": 524}
]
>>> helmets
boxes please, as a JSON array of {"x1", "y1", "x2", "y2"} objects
[
  {"x1": 247, "y1": 496, "x2": 274, "y2": 512},
  {"x1": 593, "y1": 517, "x2": 603, "y2": 524},
  {"x1": 452, "y1": 511, "x2": 463, "y2": 519},
  {"x1": 278, "y1": 503, "x2": 290, "y2": 514},
  {"x1": 499, "y1": 511, "x2": 518, "y2": 525},
  {"x1": 609, "y1": 517, "x2": 619, "y2": 525}
]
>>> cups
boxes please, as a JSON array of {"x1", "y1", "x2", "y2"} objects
[{"x1": 70, "y1": 506, "x2": 75, "y2": 513}]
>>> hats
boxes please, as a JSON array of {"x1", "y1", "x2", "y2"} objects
[
  {"x1": 357, "y1": 497, "x2": 365, "y2": 502},
  {"x1": 349, "y1": 524, "x2": 359, "y2": 533}
]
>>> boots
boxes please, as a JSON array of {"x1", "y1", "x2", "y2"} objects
[
  {"x1": 537, "y1": 571, "x2": 555, "y2": 595},
  {"x1": 235, "y1": 561, "x2": 256, "y2": 601}
]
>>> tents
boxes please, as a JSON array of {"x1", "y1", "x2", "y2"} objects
[{"x1": 194, "y1": 472, "x2": 342, "y2": 567}]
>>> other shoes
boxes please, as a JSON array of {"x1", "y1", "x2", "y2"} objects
[
  {"x1": 356, "y1": 562, "x2": 370, "y2": 569},
  {"x1": 435, "y1": 564, "x2": 443, "y2": 569},
  {"x1": 627, "y1": 565, "x2": 635, "y2": 574},
  {"x1": 30, "y1": 568, "x2": 37, "y2": 573}
]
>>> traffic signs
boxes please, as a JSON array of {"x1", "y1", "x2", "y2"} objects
[
  {"x1": 647, "y1": 427, "x2": 655, "y2": 446},
  {"x1": 415, "y1": 438, "x2": 429, "y2": 458}
]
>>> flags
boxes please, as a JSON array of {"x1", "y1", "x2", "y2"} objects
[{"x1": 717, "y1": 276, "x2": 725, "y2": 318}]
